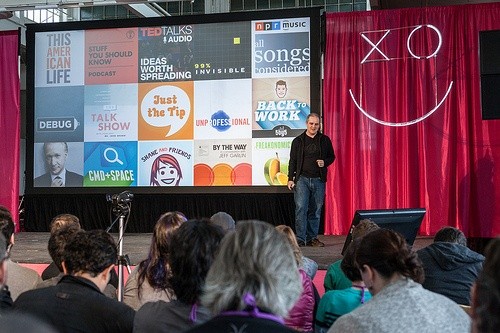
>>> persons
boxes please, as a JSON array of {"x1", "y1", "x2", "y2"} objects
[
  {"x1": 0, "y1": 205, "x2": 500, "y2": 333},
  {"x1": 288, "y1": 113, "x2": 336, "y2": 248},
  {"x1": 33, "y1": 142, "x2": 83, "y2": 187}
]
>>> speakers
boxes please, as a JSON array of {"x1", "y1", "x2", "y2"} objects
[{"x1": 479, "y1": 30, "x2": 500, "y2": 120}]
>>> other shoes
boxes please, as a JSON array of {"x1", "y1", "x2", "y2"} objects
[
  {"x1": 297, "y1": 239, "x2": 305, "y2": 247},
  {"x1": 305, "y1": 238, "x2": 326, "y2": 247}
]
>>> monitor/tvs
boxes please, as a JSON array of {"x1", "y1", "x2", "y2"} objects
[{"x1": 341, "y1": 208, "x2": 427, "y2": 256}]
